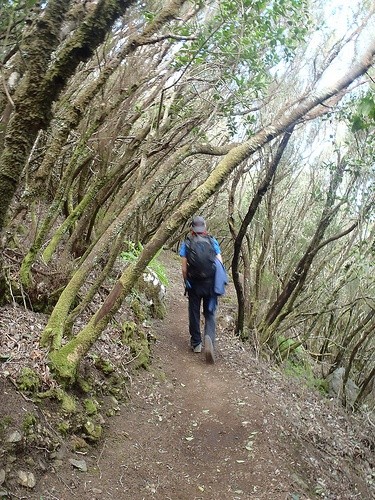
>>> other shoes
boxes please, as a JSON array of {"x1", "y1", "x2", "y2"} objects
[
  {"x1": 204, "y1": 336, "x2": 215, "y2": 364},
  {"x1": 191, "y1": 342, "x2": 200, "y2": 353}
]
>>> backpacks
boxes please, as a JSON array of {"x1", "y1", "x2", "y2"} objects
[{"x1": 188, "y1": 233, "x2": 217, "y2": 286}]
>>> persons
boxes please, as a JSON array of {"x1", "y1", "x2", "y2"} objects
[{"x1": 179, "y1": 215, "x2": 223, "y2": 364}]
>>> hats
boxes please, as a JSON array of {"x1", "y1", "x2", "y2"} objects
[{"x1": 191, "y1": 217, "x2": 207, "y2": 233}]
